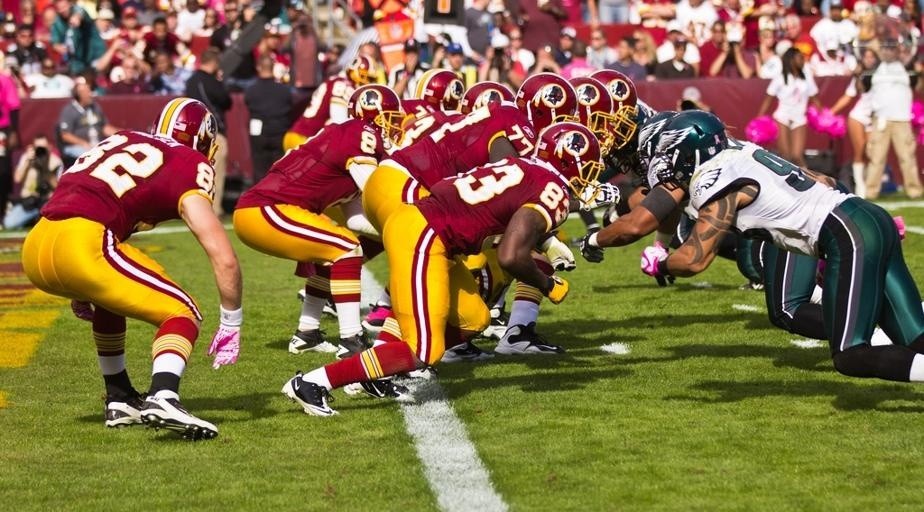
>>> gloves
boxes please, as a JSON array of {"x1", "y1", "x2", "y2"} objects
[
  {"x1": 70, "y1": 300, "x2": 94, "y2": 323},
  {"x1": 549, "y1": 278, "x2": 570, "y2": 305},
  {"x1": 210, "y1": 306, "x2": 242, "y2": 371},
  {"x1": 641, "y1": 241, "x2": 675, "y2": 288},
  {"x1": 543, "y1": 237, "x2": 576, "y2": 272},
  {"x1": 580, "y1": 231, "x2": 605, "y2": 263}
]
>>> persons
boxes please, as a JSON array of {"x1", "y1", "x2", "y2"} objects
[
  {"x1": 1, "y1": 1, "x2": 922, "y2": 230},
  {"x1": 21, "y1": 97, "x2": 241, "y2": 438},
  {"x1": 234, "y1": 71, "x2": 924, "y2": 416}
]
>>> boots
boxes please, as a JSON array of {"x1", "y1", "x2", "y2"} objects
[{"x1": 853, "y1": 163, "x2": 866, "y2": 201}]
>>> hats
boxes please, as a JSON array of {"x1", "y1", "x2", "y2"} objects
[
  {"x1": 667, "y1": 20, "x2": 688, "y2": 46},
  {"x1": 560, "y1": 26, "x2": 577, "y2": 40},
  {"x1": 98, "y1": 6, "x2": 138, "y2": 21},
  {"x1": 404, "y1": 34, "x2": 509, "y2": 55},
  {"x1": 830, "y1": 1, "x2": 844, "y2": 10}
]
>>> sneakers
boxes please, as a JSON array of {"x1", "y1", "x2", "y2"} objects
[
  {"x1": 439, "y1": 307, "x2": 563, "y2": 362},
  {"x1": 106, "y1": 393, "x2": 146, "y2": 427},
  {"x1": 738, "y1": 280, "x2": 765, "y2": 292},
  {"x1": 572, "y1": 223, "x2": 601, "y2": 247},
  {"x1": 282, "y1": 287, "x2": 437, "y2": 417},
  {"x1": 140, "y1": 389, "x2": 218, "y2": 440}
]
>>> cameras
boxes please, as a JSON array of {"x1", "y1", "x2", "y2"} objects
[
  {"x1": 33, "y1": 146, "x2": 50, "y2": 168},
  {"x1": 494, "y1": 47, "x2": 506, "y2": 63}
]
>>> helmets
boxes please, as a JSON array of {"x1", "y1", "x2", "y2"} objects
[
  {"x1": 348, "y1": 56, "x2": 517, "y2": 147},
  {"x1": 516, "y1": 69, "x2": 730, "y2": 207},
  {"x1": 152, "y1": 98, "x2": 222, "y2": 168}
]
